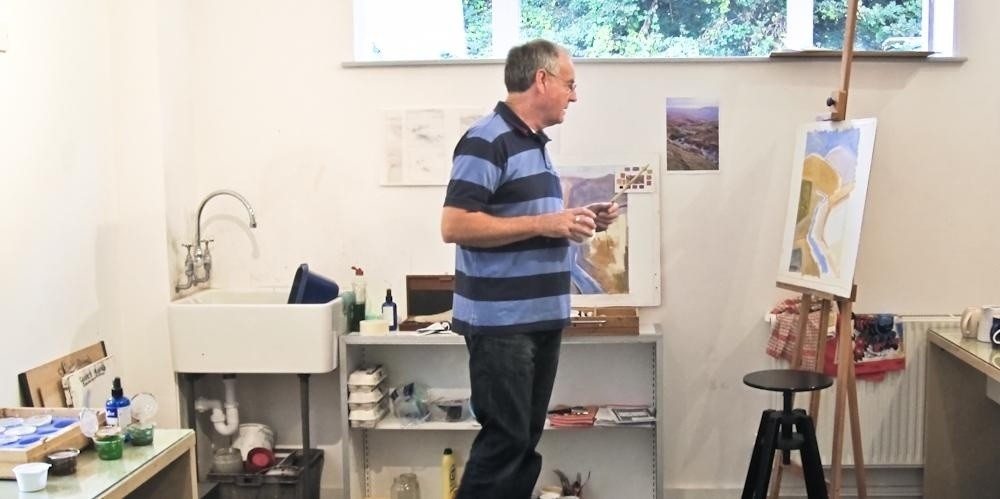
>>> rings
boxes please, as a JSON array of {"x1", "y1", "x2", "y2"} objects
[{"x1": 574, "y1": 214, "x2": 580, "y2": 224}]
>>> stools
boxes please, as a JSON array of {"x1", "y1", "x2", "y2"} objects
[{"x1": 743, "y1": 370, "x2": 837, "y2": 499}]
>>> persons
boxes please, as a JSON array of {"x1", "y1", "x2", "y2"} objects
[{"x1": 438, "y1": 37, "x2": 621, "y2": 497}]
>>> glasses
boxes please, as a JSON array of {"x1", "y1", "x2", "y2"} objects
[{"x1": 544, "y1": 68, "x2": 577, "y2": 92}]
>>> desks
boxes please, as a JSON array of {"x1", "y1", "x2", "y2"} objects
[
  {"x1": 926, "y1": 325, "x2": 1000, "y2": 498},
  {"x1": 1, "y1": 428, "x2": 198, "y2": 499}
]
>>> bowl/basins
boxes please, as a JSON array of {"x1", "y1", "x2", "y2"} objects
[{"x1": 287, "y1": 260, "x2": 340, "y2": 304}]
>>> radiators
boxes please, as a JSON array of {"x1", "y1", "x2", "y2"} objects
[{"x1": 763, "y1": 315, "x2": 972, "y2": 469}]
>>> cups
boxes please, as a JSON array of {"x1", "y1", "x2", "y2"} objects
[
  {"x1": 46, "y1": 446, "x2": 81, "y2": 477},
  {"x1": 960, "y1": 303, "x2": 1000, "y2": 350},
  {"x1": 126, "y1": 421, "x2": 154, "y2": 446},
  {"x1": 93, "y1": 434, "x2": 125, "y2": 460},
  {"x1": 11, "y1": 461, "x2": 52, "y2": 493}
]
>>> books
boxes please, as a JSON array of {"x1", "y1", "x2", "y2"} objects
[{"x1": 549, "y1": 400, "x2": 655, "y2": 429}]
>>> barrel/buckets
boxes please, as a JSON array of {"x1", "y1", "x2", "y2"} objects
[{"x1": 230, "y1": 424, "x2": 276, "y2": 460}]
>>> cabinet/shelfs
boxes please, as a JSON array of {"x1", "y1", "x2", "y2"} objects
[{"x1": 341, "y1": 325, "x2": 658, "y2": 499}]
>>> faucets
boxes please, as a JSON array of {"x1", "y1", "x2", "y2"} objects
[{"x1": 175, "y1": 189, "x2": 258, "y2": 292}]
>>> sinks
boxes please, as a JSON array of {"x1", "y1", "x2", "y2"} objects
[{"x1": 168, "y1": 286, "x2": 350, "y2": 373}]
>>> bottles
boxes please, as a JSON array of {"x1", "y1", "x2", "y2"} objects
[
  {"x1": 441, "y1": 446, "x2": 457, "y2": 499},
  {"x1": 390, "y1": 471, "x2": 422, "y2": 499},
  {"x1": 350, "y1": 265, "x2": 367, "y2": 305},
  {"x1": 380, "y1": 288, "x2": 398, "y2": 331}
]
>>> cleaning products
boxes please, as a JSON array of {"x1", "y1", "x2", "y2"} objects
[{"x1": 351, "y1": 265, "x2": 367, "y2": 332}]
